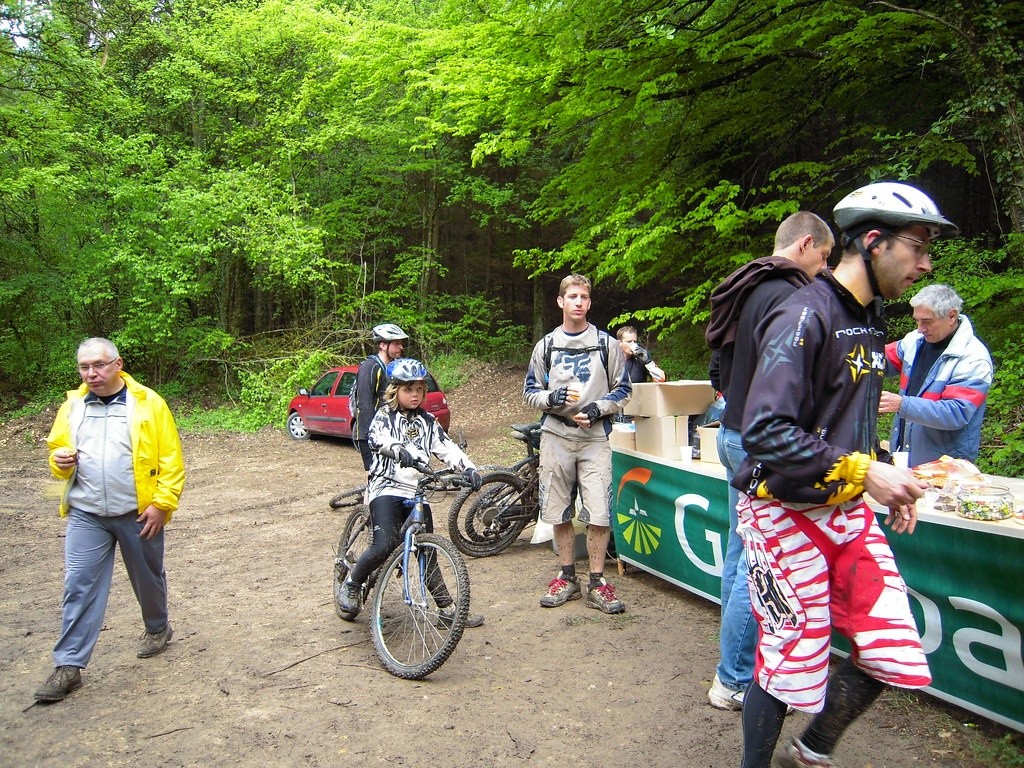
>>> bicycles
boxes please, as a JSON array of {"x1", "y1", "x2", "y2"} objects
[
  {"x1": 328, "y1": 440, "x2": 467, "y2": 508},
  {"x1": 332, "y1": 448, "x2": 472, "y2": 679},
  {"x1": 448, "y1": 422, "x2": 542, "y2": 558}
]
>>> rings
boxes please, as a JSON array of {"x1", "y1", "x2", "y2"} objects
[{"x1": 895, "y1": 511, "x2": 901, "y2": 517}]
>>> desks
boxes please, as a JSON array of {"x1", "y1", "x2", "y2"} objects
[{"x1": 612, "y1": 446, "x2": 1023, "y2": 733}]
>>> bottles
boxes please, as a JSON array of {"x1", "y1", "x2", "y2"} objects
[{"x1": 955, "y1": 482, "x2": 1015, "y2": 521}]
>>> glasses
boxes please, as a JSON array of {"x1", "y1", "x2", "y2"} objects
[
  {"x1": 78, "y1": 357, "x2": 117, "y2": 373},
  {"x1": 862, "y1": 229, "x2": 930, "y2": 261}
]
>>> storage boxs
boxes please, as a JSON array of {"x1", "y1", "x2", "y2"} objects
[{"x1": 610, "y1": 380, "x2": 721, "y2": 461}]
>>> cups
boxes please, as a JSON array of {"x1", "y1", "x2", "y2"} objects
[
  {"x1": 569, "y1": 382, "x2": 584, "y2": 401},
  {"x1": 892, "y1": 451, "x2": 908, "y2": 470},
  {"x1": 628, "y1": 341, "x2": 638, "y2": 353},
  {"x1": 63, "y1": 445, "x2": 78, "y2": 466}
]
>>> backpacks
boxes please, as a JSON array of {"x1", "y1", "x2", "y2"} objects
[{"x1": 348, "y1": 355, "x2": 387, "y2": 417}]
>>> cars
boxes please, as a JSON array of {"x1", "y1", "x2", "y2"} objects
[{"x1": 286, "y1": 366, "x2": 450, "y2": 453}]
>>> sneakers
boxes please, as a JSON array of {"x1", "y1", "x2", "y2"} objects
[
  {"x1": 34, "y1": 666, "x2": 83, "y2": 700},
  {"x1": 540, "y1": 570, "x2": 582, "y2": 607},
  {"x1": 585, "y1": 577, "x2": 626, "y2": 613},
  {"x1": 338, "y1": 574, "x2": 363, "y2": 613},
  {"x1": 136, "y1": 621, "x2": 173, "y2": 657},
  {"x1": 707, "y1": 673, "x2": 793, "y2": 716},
  {"x1": 772, "y1": 736, "x2": 834, "y2": 768},
  {"x1": 437, "y1": 603, "x2": 485, "y2": 629}
]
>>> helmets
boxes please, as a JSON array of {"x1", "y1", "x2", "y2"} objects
[
  {"x1": 373, "y1": 324, "x2": 409, "y2": 345},
  {"x1": 386, "y1": 358, "x2": 427, "y2": 385},
  {"x1": 833, "y1": 182, "x2": 959, "y2": 233}
]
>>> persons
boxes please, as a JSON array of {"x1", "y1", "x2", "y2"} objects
[
  {"x1": 336, "y1": 358, "x2": 485, "y2": 631},
  {"x1": 613, "y1": 326, "x2": 666, "y2": 423},
  {"x1": 704, "y1": 211, "x2": 835, "y2": 714},
  {"x1": 33, "y1": 338, "x2": 186, "y2": 702},
  {"x1": 876, "y1": 284, "x2": 992, "y2": 468},
  {"x1": 729, "y1": 182, "x2": 961, "y2": 768},
  {"x1": 521, "y1": 273, "x2": 632, "y2": 613},
  {"x1": 356, "y1": 324, "x2": 409, "y2": 472}
]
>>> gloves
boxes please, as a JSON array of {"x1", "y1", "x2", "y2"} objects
[
  {"x1": 390, "y1": 443, "x2": 414, "y2": 469},
  {"x1": 634, "y1": 346, "x2": 648, "y2": 362},
  {"x1": 462, "y1": 468, "x2": 483, "y2": 492}
]
